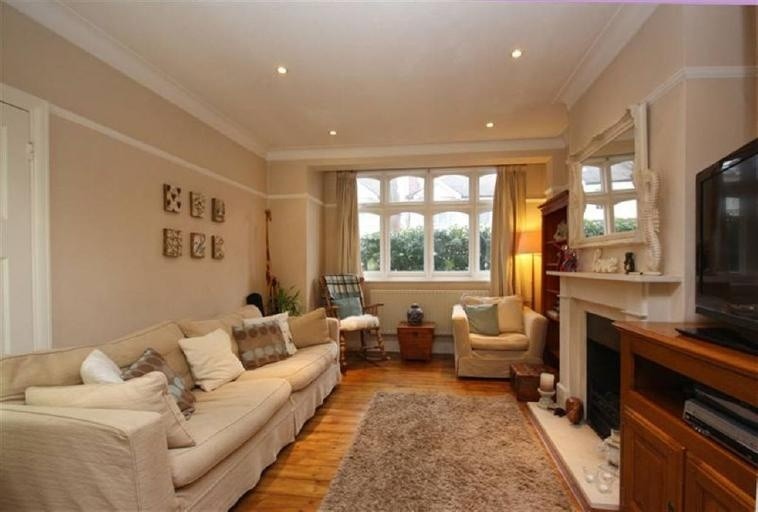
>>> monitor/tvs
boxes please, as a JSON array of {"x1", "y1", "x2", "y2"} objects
[{"x1": 674, "y1": 138, "x2": 758, "y2": 355}]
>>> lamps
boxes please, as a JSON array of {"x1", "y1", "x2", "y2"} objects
[{"x1": 515, "y1": 229, "x2": 541, "y2": 312}]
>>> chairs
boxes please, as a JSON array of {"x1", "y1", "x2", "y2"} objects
[{"x1": 315, "y1": 266, "x2": 390, "y2": 371}]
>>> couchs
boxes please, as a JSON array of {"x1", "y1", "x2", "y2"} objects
[
  {"x1": 0, "y1": 303, "x2": 341, "y2": 511},
  {"x1": 448, "y1": 289, "x2": 549, "y2": 382}
]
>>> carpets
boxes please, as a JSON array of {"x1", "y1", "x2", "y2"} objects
[{"x1": 315, "y1": 388, "x2": 577, "y2": 511}]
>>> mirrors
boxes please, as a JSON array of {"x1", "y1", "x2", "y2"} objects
[{"x1": 563, "y1": 97, "x2": 653, "y2": 250}]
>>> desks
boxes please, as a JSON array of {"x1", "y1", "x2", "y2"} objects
[{"x1": 395, "y1": 319, "x2": 433, "y2": 363}]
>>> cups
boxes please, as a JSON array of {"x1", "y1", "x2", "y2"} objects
[{"x1": 583, "y1": 464, "x2": 615, "y2": 493}]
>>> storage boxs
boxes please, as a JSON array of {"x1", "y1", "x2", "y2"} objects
[{"x1": 506, "y1": 361, "x2": 556, "y2": 405}]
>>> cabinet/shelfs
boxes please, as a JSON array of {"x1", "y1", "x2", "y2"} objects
[
  {"x1": 536, "y1": 188, "x2": 568, "y2": 369},
  {"x1": 610, "y1": 317, "x2": 755, "y2": 511}
]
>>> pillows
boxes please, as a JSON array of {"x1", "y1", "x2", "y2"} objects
[
  {"x1": 240, "y1": 310, "x2": 298, "y2": 360},
  {"x1": 286, "y1": 307, "x2": 331, "y2": 349},
  {"x1": 179, "y1": 327, "x2": 246, "y2": 393},
  {"x1": 232, "y1": 320, "x2": 288, "y2": 374},
  {"x1": 465, "y1": 302, "x2": 498, "y2": 336},
  {"x1": 21, "y1": 369, "x2": 199, "y2": 450},
  {"x1": 121, "y1": 346, "x2": 194, "y2": 422},
  {"x1": 333, "y1": 292, "x2": 366, "y2": 320},
  {"x1": 77, "y1": 346, "x2": 121, "y2": 385},
  {"x1": 480, "y1": 295, "x2": 524, "y2": 334}
]
